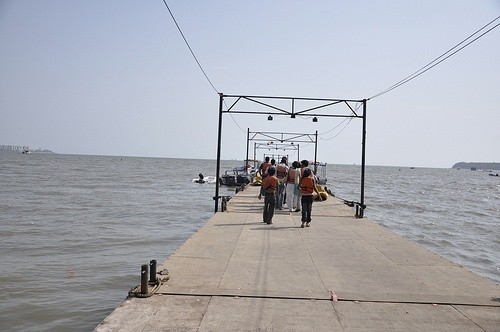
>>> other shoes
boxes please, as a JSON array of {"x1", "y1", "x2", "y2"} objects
[
  {"x1": 306, "y1": 223, "x2": 310, "y2": 226},
  {"x1": 301, "y1": 223, "x2": 304, "y2": 227},
  {"x1": 264, "y1": 219, "x2": 272, "y2": 224},
  {"x1": 295, "y1": 209, "x2": 301, "y2": 212},
  {"x1": 279, "y1": 207, "x2": 284, "y2": 210}
]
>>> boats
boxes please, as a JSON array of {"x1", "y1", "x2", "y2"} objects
[
  {"x1": 314, "y1": 162, "x2": 327, "y2": 166},
  {"x1": 456, "y1": 165, "x2": 499, "y2": 176},
  {"x1": 220, "y1": 168, "x2": 250, "y2": 187}
]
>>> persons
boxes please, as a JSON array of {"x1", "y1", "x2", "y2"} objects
[
  {"x1": 262, "y1": 168, "x2": 278, "y2": 224},
  {"x1": 297, "y1": 169, "x2": 323, "y2": 227},
  {"x1": 257, "y1": 157, "x2": 317, "y2": 212},
  {"x1": 198, "y1": 173, "x2": 203, "y2": 184},
  {"x1": 245, "y1": 164, "x2": 252, "y2": 168}
]
